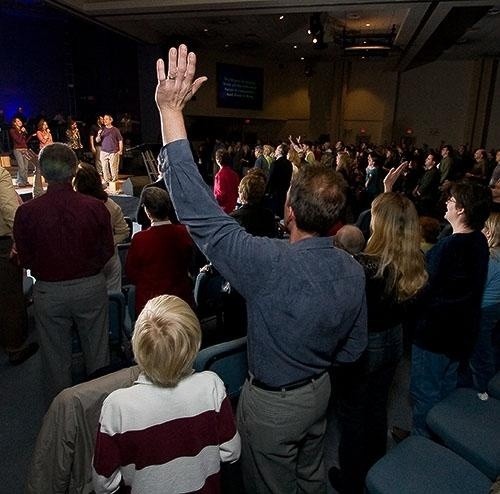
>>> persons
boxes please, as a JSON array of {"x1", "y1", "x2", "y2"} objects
[{"x1": 1, "y1": 44, "x2": 500, "y2": 493}]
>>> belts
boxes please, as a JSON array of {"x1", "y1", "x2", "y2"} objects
[{"x1": 247, "y1": 371, "x2": 329, "y2": 392}]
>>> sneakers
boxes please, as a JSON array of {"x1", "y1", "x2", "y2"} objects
[
  {"x1": 327, "y1": 464, "x2": 362, "y2": 494},
  {"x1": 17, "y1": 182, "x2": 33, "y2": 186},
  {"x1": 391, "y1": 423, "x2": 413, "y2": 443}
]
